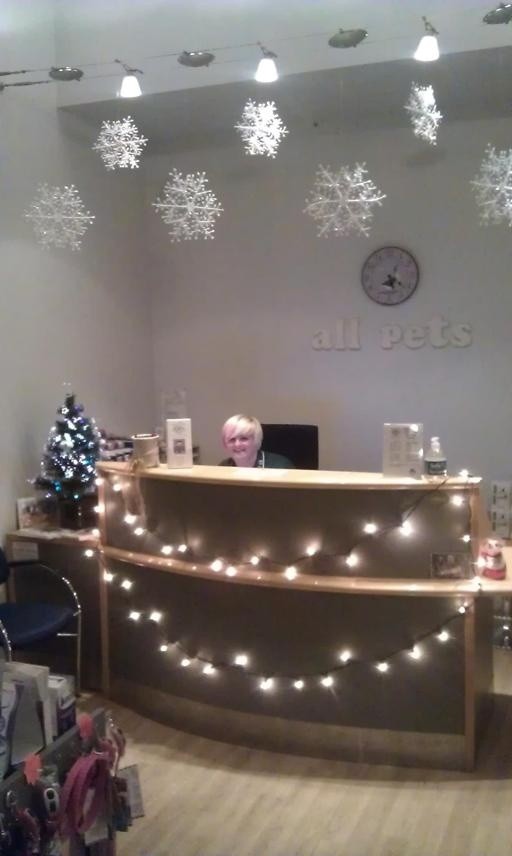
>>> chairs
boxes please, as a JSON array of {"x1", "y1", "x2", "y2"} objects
[
  {"x1": 261, "y1": 423, "x2": 320, "y2": 470},
  {"x1": 1, "y1": 542, "x2": 86, "y2": 701}
]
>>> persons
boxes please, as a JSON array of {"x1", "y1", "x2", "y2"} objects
[
  {"x1": 216, "y1": 413, "x2": 297, "y2": 470},
  {"x1": 435, "y1": 554, "x2": 463, "y2": 579}
]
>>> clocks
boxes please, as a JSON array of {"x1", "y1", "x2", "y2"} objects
[{"x1": 361, "y1": 247, "x2": 420, "y2": 304}]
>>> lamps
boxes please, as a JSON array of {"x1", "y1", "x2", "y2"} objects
[{"x1": 114, "y1": 15, "x2": 441, "y2": 104}]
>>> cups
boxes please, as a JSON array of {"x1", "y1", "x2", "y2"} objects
[{"x1": 130, "y1": 431, "x2": 161, "y2": 469}]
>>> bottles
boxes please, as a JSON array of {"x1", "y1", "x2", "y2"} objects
[{"x1": 422, "y1": 434, "x2": 450, "y2": 484}]
